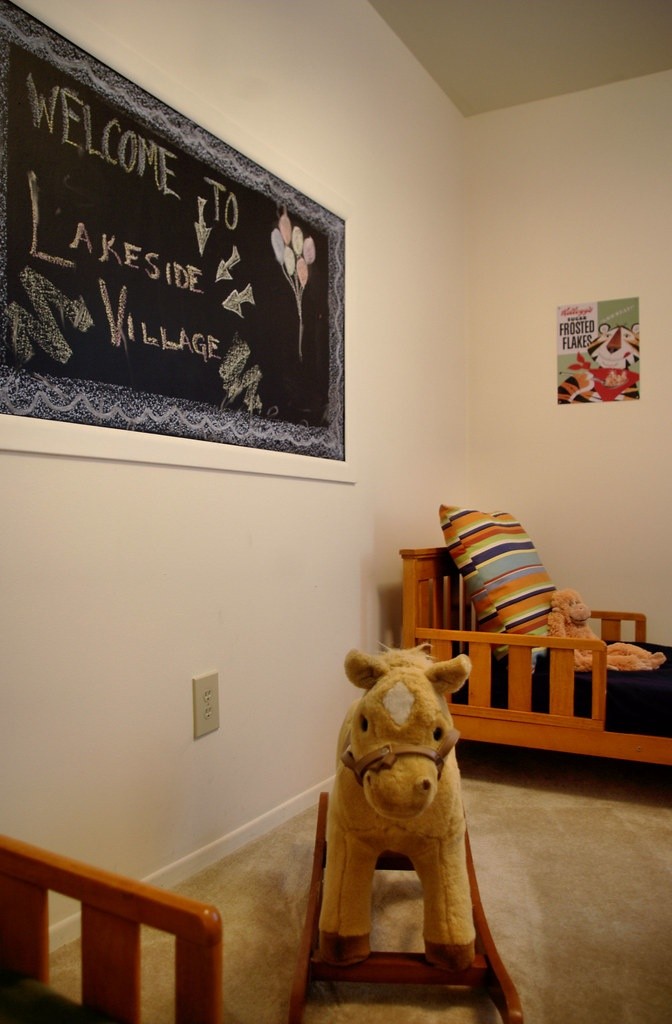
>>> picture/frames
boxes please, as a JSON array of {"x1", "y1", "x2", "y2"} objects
[{"x1": 0, "y1": 0, "x2": 356, "y2": 484}]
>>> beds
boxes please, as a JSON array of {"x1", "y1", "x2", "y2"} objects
[{"x1": 398, "y1": 548, "x2": 672, "y2": 766}]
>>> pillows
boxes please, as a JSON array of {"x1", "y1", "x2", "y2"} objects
[{"x1": 439, "y1": 504, "x2": 558, "y2": 673}]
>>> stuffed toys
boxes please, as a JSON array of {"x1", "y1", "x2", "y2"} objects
[{"x1": 547, "y1": 587, "x2": 666, "y2": 670}]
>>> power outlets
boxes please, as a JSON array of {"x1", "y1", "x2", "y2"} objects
[{"x1": 193, "y1": 671, "x2": 220, "y2": 737}]
